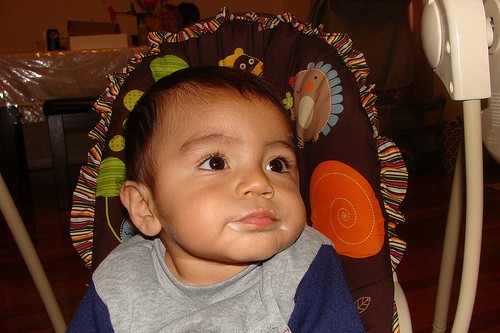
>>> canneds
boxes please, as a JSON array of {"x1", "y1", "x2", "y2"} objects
[{"x1": 46, "y1": 28, "x2": 60, "y2": 50}]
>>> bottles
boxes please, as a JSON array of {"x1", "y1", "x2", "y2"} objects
[{"x1": 46, "y1": 29, "x2": 60, "y2": 51}]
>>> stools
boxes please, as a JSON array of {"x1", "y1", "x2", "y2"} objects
[{"x1": 43, "y1": 96, "x2": 103, "y2": 212}]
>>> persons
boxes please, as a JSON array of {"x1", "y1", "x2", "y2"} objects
[{"x1": 62, "y1": 64, "x2": 368, "y2": 333}]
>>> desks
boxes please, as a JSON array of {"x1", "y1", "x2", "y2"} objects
[{"x1": 0, "y1": 47, "x2": 148, "y2": 212}]
